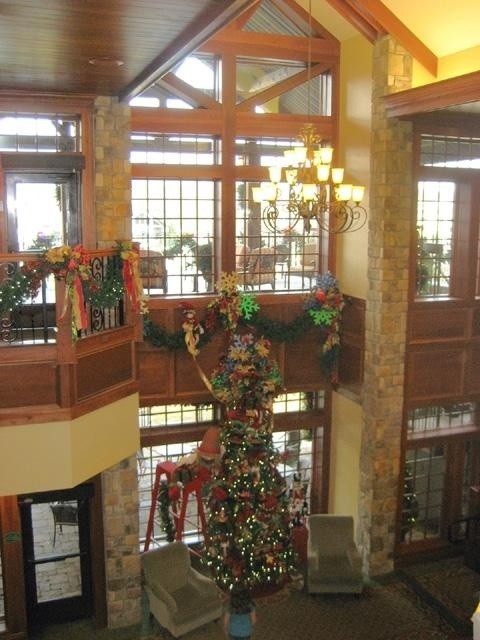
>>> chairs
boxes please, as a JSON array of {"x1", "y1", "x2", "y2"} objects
[
  {"x1": 142, "y1": 541, "x2": 224, "y2": 639},
  {"x1": 234, "y1": 243, "x2": 316, "y2": 291},
  {"x1": 305, "y1": 513, "x2": 366, "y2": 601},
  {"x1": 49, "y1": 504, "x2": 79, "y2": 547},
  {"x1": 138, "y1": 250, "x2": 169, "y2": 295}
]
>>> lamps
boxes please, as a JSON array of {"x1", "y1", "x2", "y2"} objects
[{"x1": 250, "y1": 0, "x2": 368, "y2": 236}]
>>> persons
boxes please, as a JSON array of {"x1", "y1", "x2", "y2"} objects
[
  {"x1": 197, "y1": 417, "x2": 225, "y2": 477},
  {"x1": 222, "y1": 580, "x2": 258, "y2": 640}
]
